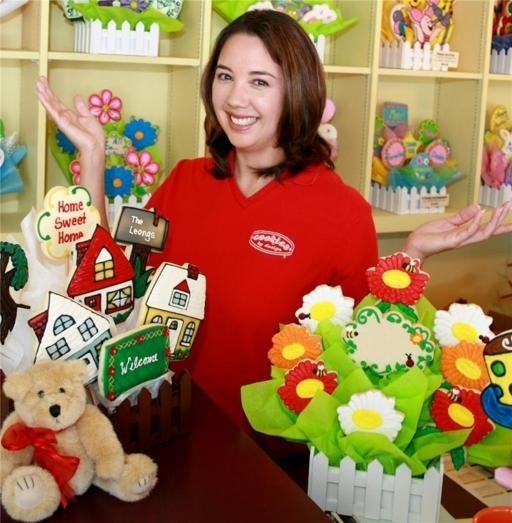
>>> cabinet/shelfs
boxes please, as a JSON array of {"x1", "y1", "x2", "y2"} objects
[{"x1": 2, "y1": 0, "x2": 511, "y2": 244}]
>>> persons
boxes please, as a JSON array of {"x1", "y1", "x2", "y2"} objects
[{"x1": 35, "y1": 7, "x2": 512, "y2": 456}]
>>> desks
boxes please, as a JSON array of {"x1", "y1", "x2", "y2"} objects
[{"x1": 1, "y1": 376, "x2": 332, "y2": 523}]
